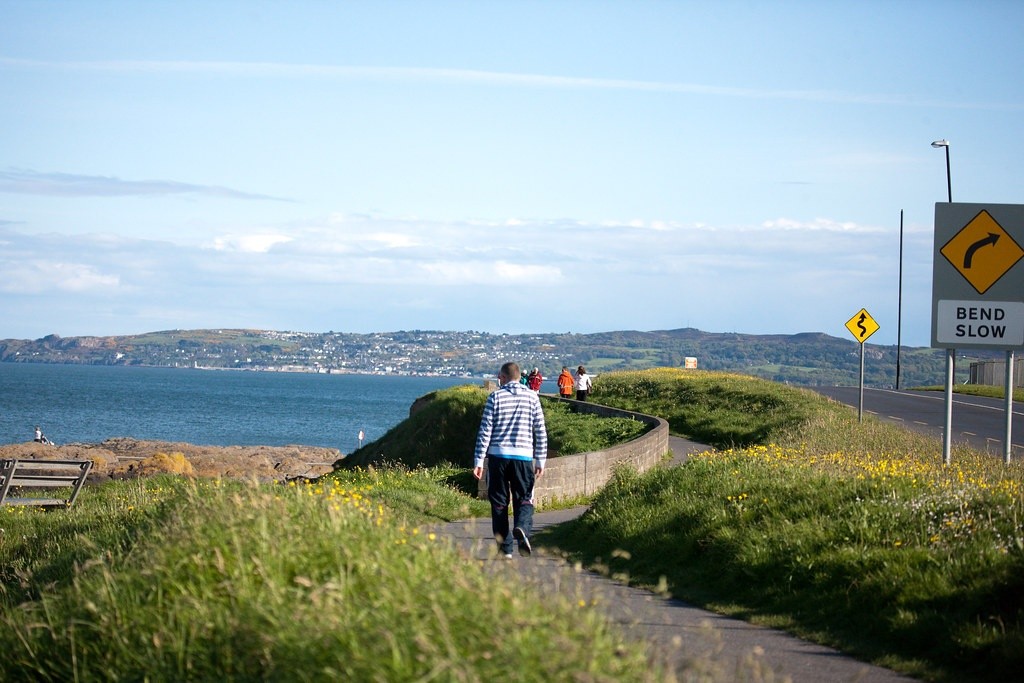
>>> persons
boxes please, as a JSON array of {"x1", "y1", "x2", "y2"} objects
[
  {"x1": 574, "y1": 366, "x2": 592, "y2": 401},
  {"x1": 521, "y1": 367, "x2": 544, "y2": 394},
  {"x1": 473, "y1": 362, "x2": 548, "y2": 558},
  {"x1": 34, "y1": 427, "x2": 50, "y2": 445},
  {"x1": 557, "y1": 366, "x2": 575, "y2": 399}
]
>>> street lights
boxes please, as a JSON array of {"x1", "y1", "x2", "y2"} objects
[{"x1": 931, "y1": 139, "x2": 952, "y2": 203}]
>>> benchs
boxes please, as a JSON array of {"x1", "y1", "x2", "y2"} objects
[{"x1": 0, "y1": 456, "x2": 95, "y2": 510}]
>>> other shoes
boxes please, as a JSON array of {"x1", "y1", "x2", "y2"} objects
[
  {"x1": 495, "y1": 551, "x2": 513, "y2": 559},
  {"x1": 513, "y1": 527, "x2": 531, "y2": 557}
]
